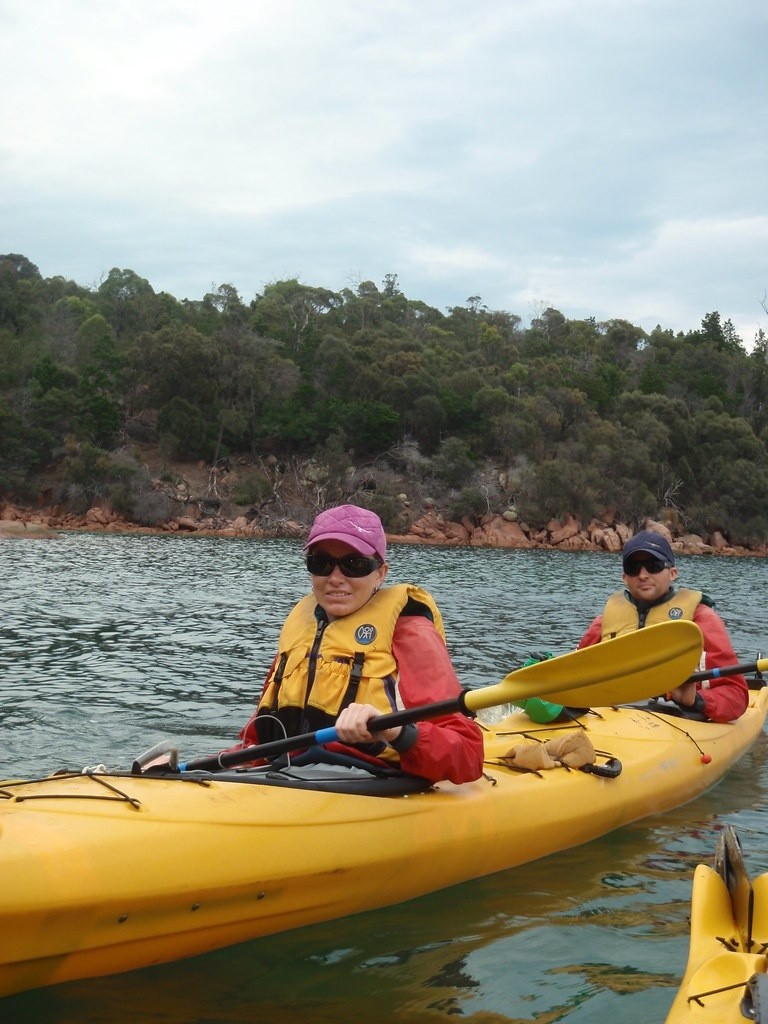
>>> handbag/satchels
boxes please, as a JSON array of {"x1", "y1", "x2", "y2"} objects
[{"x1": 510, "y1": 652, "x2": 603, "y2": 730}]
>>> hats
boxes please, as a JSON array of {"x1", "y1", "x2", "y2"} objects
[
  {"x1": 622, "y1": 531, "x2": 675, "y2": 569},
  {"x1": 303, "y1": 505, "x2": 387, "y2": 566}
]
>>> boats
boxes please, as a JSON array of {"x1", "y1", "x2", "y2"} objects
[{"x1": 0, "y1": 682, "x2": 767, "y2": 1004}]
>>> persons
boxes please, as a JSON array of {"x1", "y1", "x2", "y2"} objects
[
  {"x1": 573, "y1": 530, "x2": 750, "y2": 723},
  {"x1": 141, "y1": 504, "x2": 484, "y2": 785}
]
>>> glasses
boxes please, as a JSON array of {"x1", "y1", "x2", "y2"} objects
[
  {"x1": 623, "y1": 558, "x2": 674, "y2": 577},
  {"x1": 306, "y1": 553, "x2": 382, "y2": 578}
]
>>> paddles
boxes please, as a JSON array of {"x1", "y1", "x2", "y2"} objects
[
  {"x1": 682, "y1": 651, "x2": 768, "y2": 687},
  {"x1": 178, "y1": 615, "x2": 708, "y2": 773}
]
